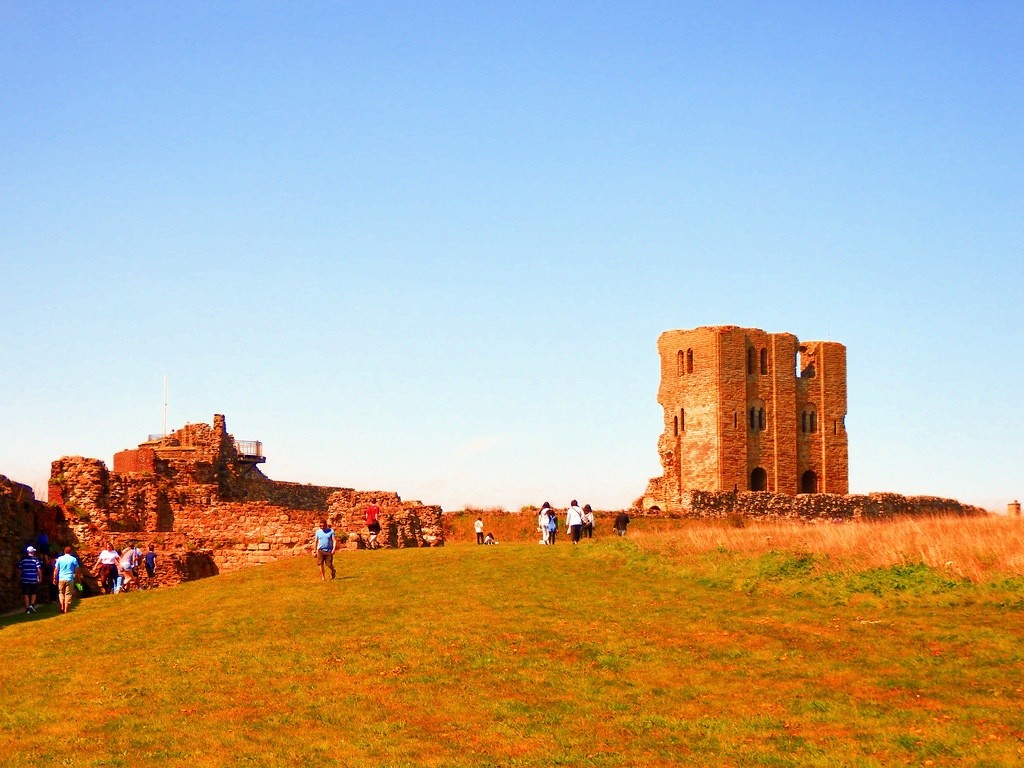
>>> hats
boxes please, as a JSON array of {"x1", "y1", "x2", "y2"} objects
[
  {"x1": 26, "y1": 546, "x2": 36, "y2": 553},
  {"x1": 64, "y1": 546, "x2": 72, "y2": 553}
]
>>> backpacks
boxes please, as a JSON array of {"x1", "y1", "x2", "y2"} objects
[{"x1": 547, "y1": 516, "x2": 556, "y2": 532}]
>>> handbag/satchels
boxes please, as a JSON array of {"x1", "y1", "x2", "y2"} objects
[{"x1": 582, "y1": 517, "x2": 592, "y2": 529}]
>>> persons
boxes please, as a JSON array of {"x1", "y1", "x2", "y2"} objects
[
  {"x1": 313, "y1": 519, "x2": 337, "y2": 582},
  {"x1": 142, "y1": 544, "x2": 157, "y2": 590},
  {"x1": 565, "y1": 499, "x2": 595, "y2": 545},
  {"x1": 365, "y1": 497, "x2": 381, "y2": 551},
  {"x1": 92, "y1": 541, "x2": 143, "y2": 595},
  {"x1": 15, "y1": 545, "x2": 42, "y2": 615},
  {"x1": 538, "y1": 501, "x2": 559, "y2": 546},
  {"x1": 474, "y1": 517, "x2": 495, "y2": 546},
  {"x1": 35, "y1": 529, "x2": 81, "y2": 614},
  {"x1": 612, "y1": 509, "x2": 630, "y2": 537}
]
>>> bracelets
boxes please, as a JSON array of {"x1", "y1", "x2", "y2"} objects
[{"x1": 313, "y1": 552, "x2": 316, "y2": 553}]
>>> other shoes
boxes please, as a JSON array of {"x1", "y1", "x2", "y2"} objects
[
  {"x1": 330, "y1": 569, "x2": 336, "y2": 579},
  {"x1": 25, "y1": 610, "x2": 30, "y2": 614},
  {"x1": 29, "y1": 605, "x2": 37, "y2": 613}
]
